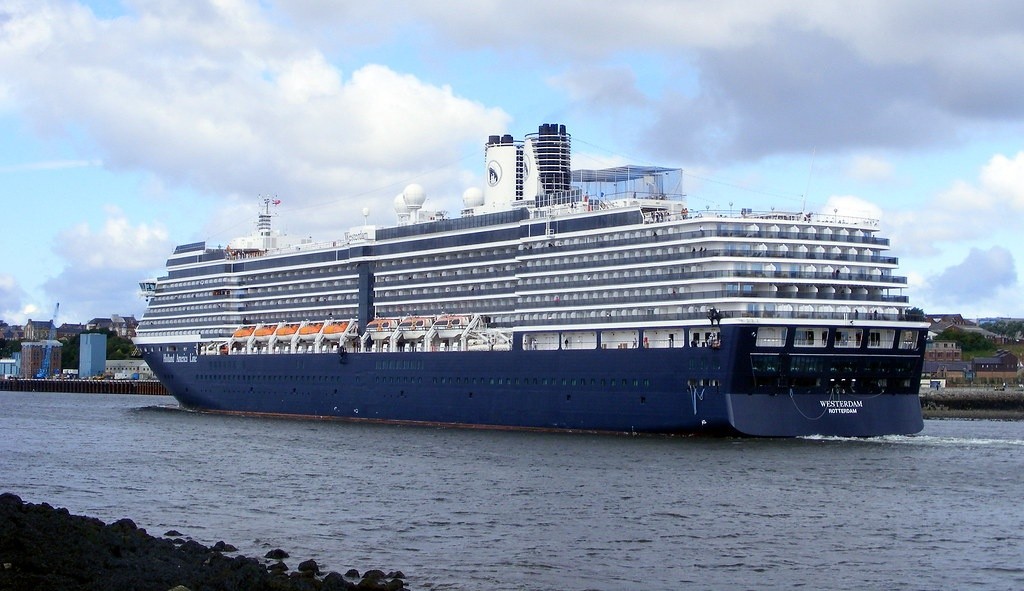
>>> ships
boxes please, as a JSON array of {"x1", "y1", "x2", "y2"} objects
[{"x1": 133, "y1": 122, "x2": 934, "y2": 442}]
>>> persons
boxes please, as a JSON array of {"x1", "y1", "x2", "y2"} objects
[
  {"x1": 836, "y1": 269, "x2": 840, "y2": 279},
  {"x1": 587, "y1": 276, "x2": 591, "y2": 280},
  {"x1": 692, "y1": 247, "x2": 695, "y2": 257},
  {"x1": 854, "y1": 310, "x2": 858, "y2": 319},
  {"x1": 606, "y1": 312, "x2": 609, "y2": 319},
  {"x1": 653, "y1": 231, "x2": 657, "y2": 240},
  {"x1": 633, "y1": 192, "x2": 637, "y2": 198},
  {"x1": 555, "y1": 297, "x2": 559, "y2": 301},
  {"x1": 644, "y1": 336, "x2": 648, "y2": 344},
  {"x1": 936, "y1": 385, "x2": 938, "y2": 390},
  {"x1": 874, "y1": 310, "x2": 878, "y2": 319},
  {"x1": 681, "y1": 208, "x2": 688, "y2": 219},
  {"x1": 565, "y1": 339, "x2": 568, "y2": 347},
  {"x1": 872, "y1": 235, "x2": 876, "y2": 241},
  {"x1": 700, "y1": 247, "x2": 707, "y2": 257},
  {"x1": 632, "y1": 338, "x2": 637, "y2": 348},
  {"x1": 549, "y1": 243, "x2": 555, "y2": 247},
  {"x1": 524, "y1": 247, "x2": 527, "y2": 250},
  {"x1": 699, "y1": 226, "x2": 704, "y2": 236}
]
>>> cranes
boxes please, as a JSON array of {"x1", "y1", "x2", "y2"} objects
[{"x1": 36, "y1": 302, "x2": 61, "y2": 379}]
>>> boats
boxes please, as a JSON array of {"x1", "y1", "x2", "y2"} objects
[
  {"x1": 432, "y1": 314, "x2": 470, "y2": 339},
  {"x1": 254, "y1": 323, "x2": 278, "y2": 337},
  {"x1": 233, "y1": 325, "x2": 257, "y2": 338},
  {"x1": 322, "y1": 320, "x2": 349, "y2": 333},
  {"x1": 274, "y1": 322, "x2": 300, "y2": 336},
  {"x1": 397, "y1": 315, "x2": 434, "y2": 332},
  {"x1": 365, "y1": 318, "x2": 399, "y2": 332},
  {"x1": 297, "y1": 321, "x2": 325, "y2": 335}
]
